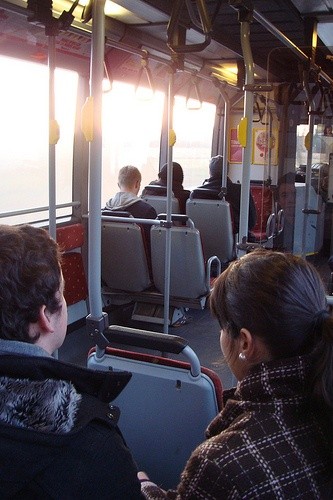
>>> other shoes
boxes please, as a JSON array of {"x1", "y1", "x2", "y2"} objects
[{"x1": 171, "y1": 314, "x2": 193, "y2": 328}]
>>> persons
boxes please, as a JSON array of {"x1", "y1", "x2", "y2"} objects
[
  {"x1": 265, "y1": 172, "x2": 325, "y2": 257},
  {"x1": 137, "y1": 251, "x2": 333, "y2": 500},
  {"x1": 325, "y1": 126, "x2": 333, "y2": 137},
  {"x1": 0, "y1": 225, "x2": 142, "y2": 500},
  {"x1": 101, "y1": 155, "x2": 257, "y2": 286}
]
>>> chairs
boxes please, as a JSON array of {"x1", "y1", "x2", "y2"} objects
[
  {"x1": 86, "y1": 326, "x2": 224, "y2": 491},
  {"x1": 101, "y1": 185, "x2": 239, "y2": 300}
]
